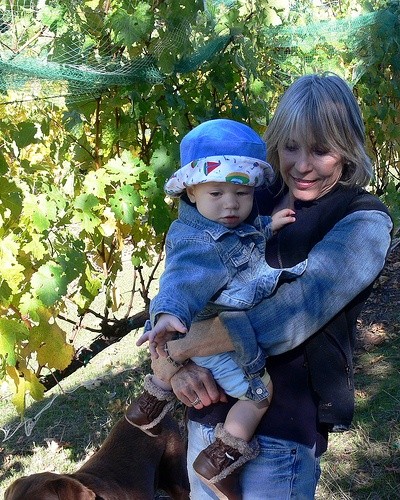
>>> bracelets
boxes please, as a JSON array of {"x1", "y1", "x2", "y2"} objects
[{"x1": 163, "y1": 343, "x2": 189, "y2": 368}]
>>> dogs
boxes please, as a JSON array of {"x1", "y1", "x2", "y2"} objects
[{"x1": 4, "y1": 390, "x2": 190, "y2": 500}]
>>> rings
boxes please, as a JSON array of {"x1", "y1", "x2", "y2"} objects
[{"x1": 191, "y1": 397, "x2": 200, "y2": 407}]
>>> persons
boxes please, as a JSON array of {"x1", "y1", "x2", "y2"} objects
[
  {"x1": 151, "y1": 72, "x2": 394, "y2": 500},
  {"x1": 136, "y1": 119, "x2": 295, "y2": 500},
  {"x1": 5, "y1": 374, "x2": 191, "y2": 500}
]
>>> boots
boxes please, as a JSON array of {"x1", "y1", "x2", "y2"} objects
[
  {"x1": 125, "y1": 373, "x2": 176, "y2": 436},
  {"x1": 191, "y1": 423, "x2": 259, "y2": 500}
]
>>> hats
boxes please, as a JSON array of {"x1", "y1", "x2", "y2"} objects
[{"x1": 163, "y1": 119, "x2": 275, "y2": 198}]
